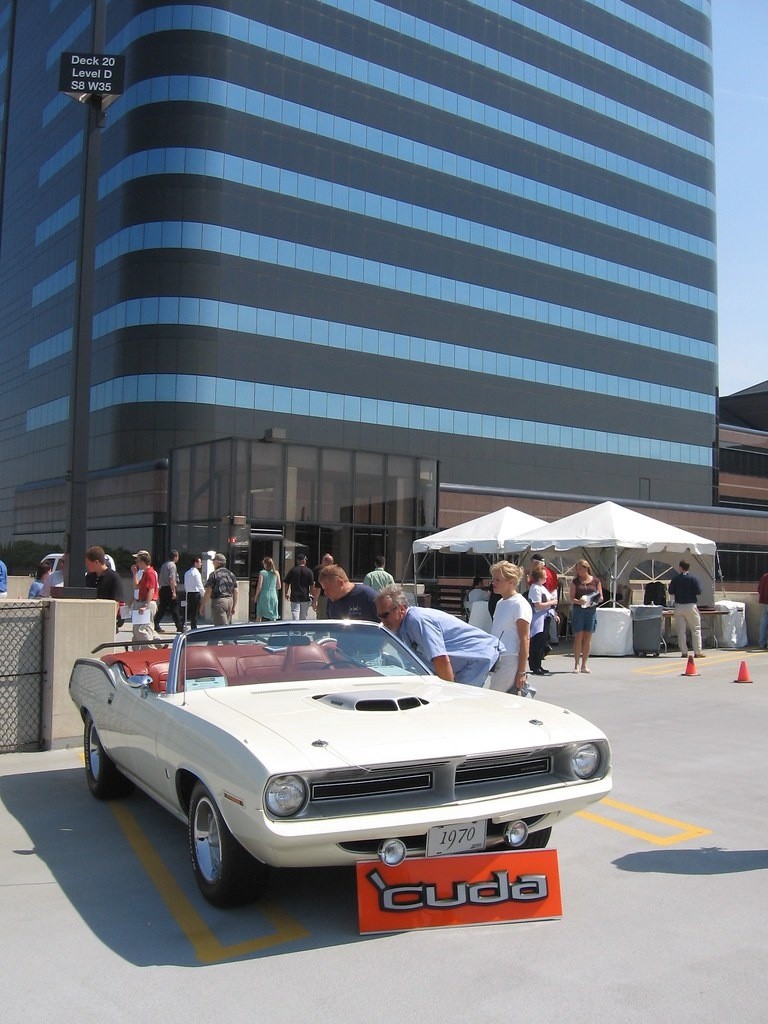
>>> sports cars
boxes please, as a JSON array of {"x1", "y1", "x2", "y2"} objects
[{"x1": 69, "y1": 619, "x2": 614, "y2": 916}]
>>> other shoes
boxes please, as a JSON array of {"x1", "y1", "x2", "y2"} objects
[
  {"x1": 541, "y1": 668, "x2": 549, "y2": 673},
  {"x1": 550, "y1": 642, "x2": 558, "y2": 645},
  {"x1": 574, "y1": 669, "x2": 579, "y2": 673},
  {"x1": 156, "y1": 628, "x2": 165, "y2": 632},
  {"x1": 682, "y1": 653, "x2": 688, "y2": 657},
  {"x1": 581, "y1": 668, "x2": 590, "y2": 673},
  {"x1": 694, "y1": 654, "x2": 705, "y2": 657},
  {"x1": 534, "y1": 669, "x2": 544, "y2": 674}
]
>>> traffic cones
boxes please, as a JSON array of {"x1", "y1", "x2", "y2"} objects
[
  {"x1": 733, "y1": 660, "x2": 754, "y2": 684},
  {"x1": 681, "y1": 655, "x2": 702, "y2": 676}
]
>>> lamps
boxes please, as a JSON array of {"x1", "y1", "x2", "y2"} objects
[
  {"x1": 264, "y1": 428, "x2": 286, "y2": 442},
  {"x1": 231, "y1": 515, "x2": 247, "y2": 526}
]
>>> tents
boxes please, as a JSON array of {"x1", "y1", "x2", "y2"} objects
[
  {"x1": 504, "y1": 500, "x2": 716, "y2": 608},
  {"x1": 413, "y1": 506, "x2": 550, "y2": 602}
]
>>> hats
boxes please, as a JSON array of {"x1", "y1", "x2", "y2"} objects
[
  {"x1": 297, "y1": 554, "x2": 309, "y2": 560},
  {"x1": 532, "y1": 554, "x2": 547, "y2": 561},
  {"x1": 211, "y1": 553, "x2": 225, "y2": 561},
  {"x1": 133, "y1": 550, "x2": 149, "y2": 557}
]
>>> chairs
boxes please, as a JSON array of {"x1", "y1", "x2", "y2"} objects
[
  {"x1": 148, "y1": 659, "x2": 171, "y2": 693},
  {"x1": 177, "y1": 646, "x2": 228, "y2": 690},
  {"x1": 283, "y1": 642, "x2": 335, "y2": 671}
]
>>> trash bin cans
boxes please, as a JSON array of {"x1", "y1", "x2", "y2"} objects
[
  {"x1": 177, "y1": 591, "x2": 185, "y2": 620},
  {"x1": 629, "y1": 604, "x2": 663, "y2": 657}
]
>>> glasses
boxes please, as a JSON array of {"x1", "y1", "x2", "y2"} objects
[{"x1": 377, "y1": 606, "x2": 399, "y2": 619}]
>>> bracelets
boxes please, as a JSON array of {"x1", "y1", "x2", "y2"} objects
[{"x1": 518, "y1": 671, "x2": 525, "y2": 675}]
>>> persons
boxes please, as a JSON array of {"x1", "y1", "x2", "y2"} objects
[
  {"x1": 526, "y1": 553, "x2": 559, "y2": 645},
  {"x1": 184, "y1": 556, "x2": 206, "y2": 629},
  {"x1": 376, "y1": 586, "x2": 506, "y2": 688},
  {"x1": 313, "y1": 554, "x2": 334, "y2": 619},
  {"x1": 758, "y1": 573, "x2": 768, "y2": 648},
  {"x1": 569, "y1": 559, "x2": 603, "y2": 674},
  {"x1": 39, "y1": 554, "x2": 65, "y2": 598},
  {"x1": 0, "y1": 560, "x2": 8, "y2": 599},
  {"x1": 199, "y1": 554, "x2": 239, "y2": 646},
  {"x1": 319, "y1": 564, "x2": 384, "y2": 667},
  {"x1": 154, "y1": 550, "x2": 188, "y2": 632},
  {"x1": 363, "y1": 556, "x2": 394, "y2": 591},
  {"x1": 29, "y1": 560, "x2": 51, "y2": 598},
  {"x1": 669, "y1": 559, "x2": 706, "y2": 658},
  {"x1": 490, "y1": 561, "x2": 532, "y2": 693},
  {"x1": 131, "y1": 550, "x2": 169, "y2": 651},
  {"x1": 255, "y1": 557, "x2": 282, "y2": 622},
  {"x1": 527, "y1": 565, "x2": 561, "y2": 675},
  {"x1": 466, "y1": 577, "x2": 483, "y2": 601},
  {"x1": 284, "y1": 554, "x2": 314, "y2": 620},
  {"x1": 86, "y1": 546, "x2": 124, "y2": 615},
  {"x1": 487, "y1": 584, "x2": 502, "y2": 622}
]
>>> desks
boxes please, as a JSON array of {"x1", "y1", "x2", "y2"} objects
[{"x1": 660, "y1": 609, "x2": 730, "y2": 654}]
